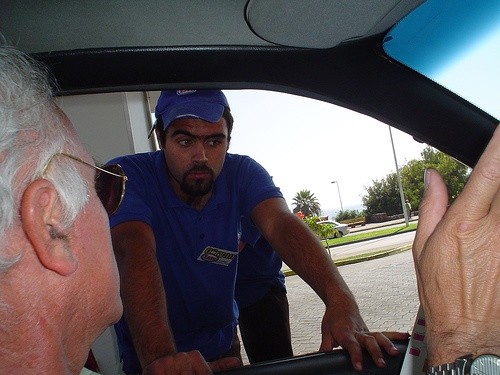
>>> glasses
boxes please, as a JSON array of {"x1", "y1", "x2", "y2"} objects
[{"x1": 41, "y1": 151, "x2": 128, "y2": 218}]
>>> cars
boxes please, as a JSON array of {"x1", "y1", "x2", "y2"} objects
[{"x1": 316, "y1": 220, "x2": 350, "y2": 238}]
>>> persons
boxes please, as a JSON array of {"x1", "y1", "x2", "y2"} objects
[
  {"x1": 405, "y1": 200, "x2": 412, "y2": 220},
  {"x1": 0, "y1": 32, "x2": 500, "y2": 374},
  {"x1": 105, "y1": 89, "x2": 410, "y2": 375}
]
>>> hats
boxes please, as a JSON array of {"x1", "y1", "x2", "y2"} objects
[{"x1": 154, "y1": 90, "x2": 230, "y2": 132}]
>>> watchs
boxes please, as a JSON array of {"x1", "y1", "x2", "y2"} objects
[{"x1": 428, "y1": 353, "x2": 499, "y2": 375}]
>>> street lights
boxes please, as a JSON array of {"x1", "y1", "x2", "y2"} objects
[{"x1": 331, "y1": 180, "x2": 344, "y2": 213}]
